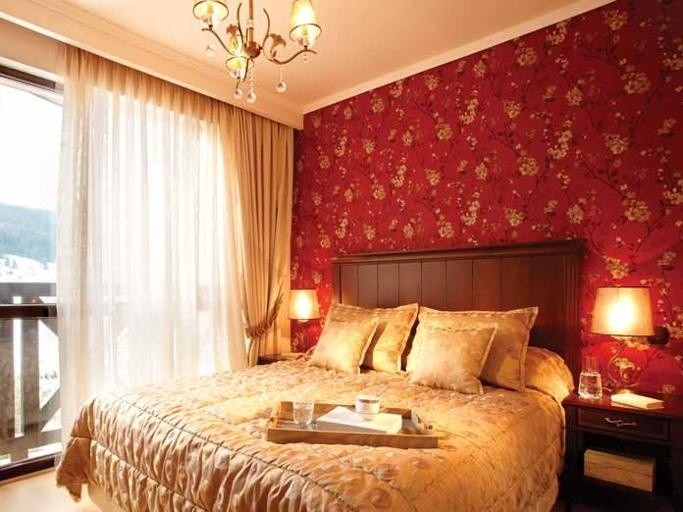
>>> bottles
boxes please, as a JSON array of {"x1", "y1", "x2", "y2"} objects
[{"x1": 578, "y1": 371, "x2": 603, "y2": 400}]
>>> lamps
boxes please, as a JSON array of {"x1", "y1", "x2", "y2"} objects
[
  {"x1": 191, "y1": 0, "x2": 322, "y2": 104},
  {"x1": 589, "y1": 287, "x2": 670, "y2": 344},
  {"x1": 288, "y1": 289, "x2": 320, "y2": 323}
]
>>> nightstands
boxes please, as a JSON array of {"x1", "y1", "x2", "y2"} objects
[
  {"x1": 561, "y1": 387, "x2": 682, "y2": 511},
  {"x1": 257, "y1": 353, "x2": 293, "y2": 365}
]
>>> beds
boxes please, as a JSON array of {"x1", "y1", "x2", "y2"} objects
[{"x1": 85, "y1": 238, "x2": 584, "y2": 511}]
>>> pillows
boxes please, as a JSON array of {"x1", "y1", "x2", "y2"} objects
[
  {"x1": 308, "y1": 302, "x2": 419, "y2": 377},
  {"x1": 405, "y1": 305, "x2": 539, "y2": 395}
]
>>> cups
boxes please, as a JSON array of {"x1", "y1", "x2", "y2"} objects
[
  {"x1": 292, "y1": 397, "x2": 314, "y2": 428},
  {"x1": 355, "y1": 396, "x2": 381, "y2": 422}
]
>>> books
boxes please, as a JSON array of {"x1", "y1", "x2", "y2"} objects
[
  {"x1": 610, "y1": 393, "x2": 665, "y2": 410},
  {"x1": 316, "y1": 406, "x2": 403, "y2": 434}
]
